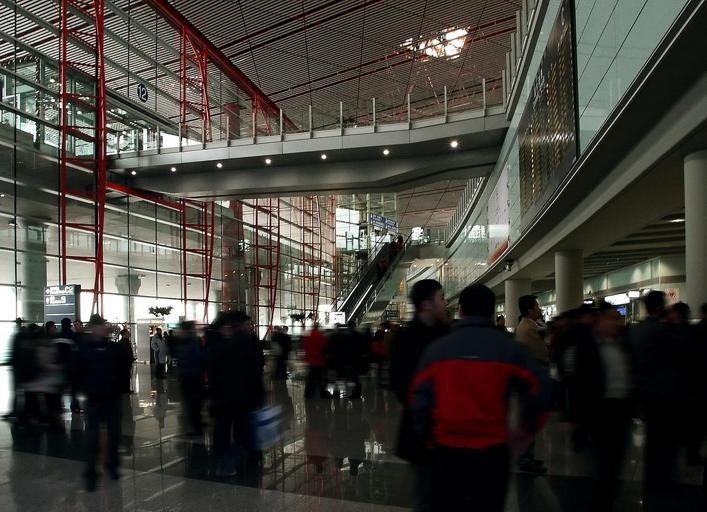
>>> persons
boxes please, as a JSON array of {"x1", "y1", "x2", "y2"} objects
[{"x1": 374, "y1": 233, "x2": 404, "y2": 281}]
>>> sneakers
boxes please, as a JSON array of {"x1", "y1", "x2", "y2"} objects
[
  {"x1": 84, "y1": 468, "x2": 96, "y2": 491},
  {"x1": 106, "y1": 465, "x2": 118, "y2": 480}
]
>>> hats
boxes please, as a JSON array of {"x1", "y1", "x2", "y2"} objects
[{"x1": 89, "y1": 313, "x2": 107, "y2": 324}]
